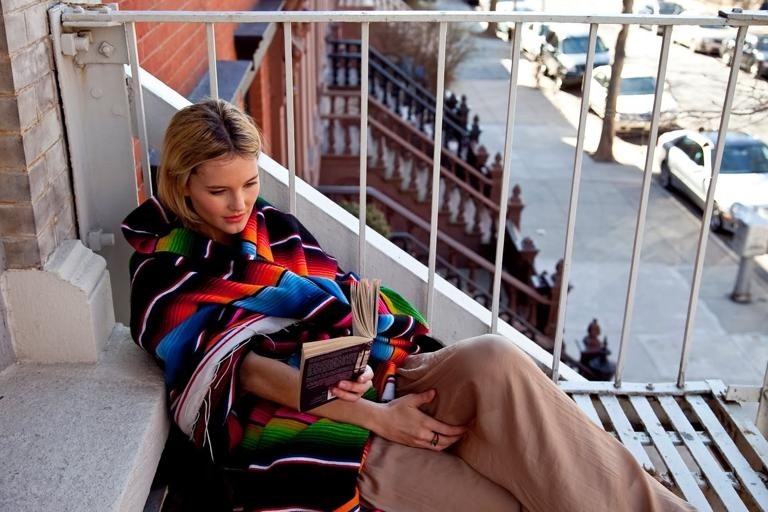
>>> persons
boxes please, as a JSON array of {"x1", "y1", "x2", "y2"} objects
[{"x1": 118, "y1": 99, "x2": 699, "y2": 512}]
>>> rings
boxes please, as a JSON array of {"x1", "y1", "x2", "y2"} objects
[{"x1": 431, "y1": 432, "x2": 439, "y2": 447}]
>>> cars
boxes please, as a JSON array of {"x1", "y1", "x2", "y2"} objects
[
  {"x1": 722, "y1": 28, "x2": 768, "y2": 80},
  {"x1": 520, "y1": 23, "x2": 612, "y2": 91},
  {"x1": 580, "y1": 64, "x2": 680, "y2": 139},
  {"x1": 672, "y1": 9, "x2": 731, "y2": 56},
  {"x1": 653, "y1": 127, "x2": 768, "y2": 236},
  {"x1": 635, "y1": 1, "x2": 684, "y2": 36}
]
